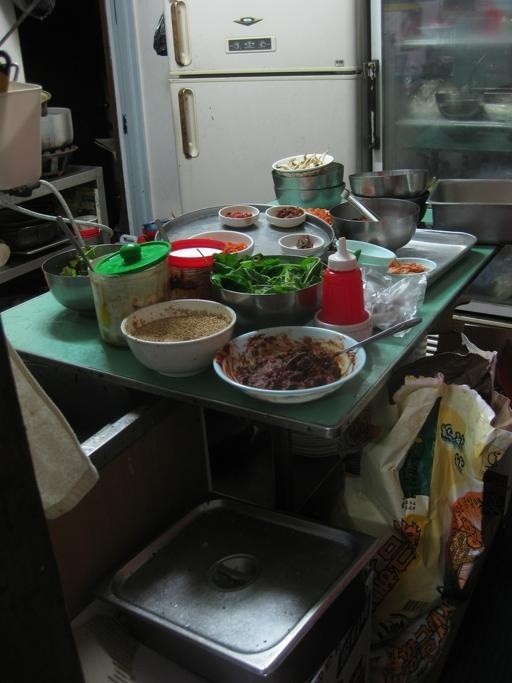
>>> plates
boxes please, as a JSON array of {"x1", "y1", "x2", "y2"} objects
[
  {"x1": 1, "y1": 244, "x2": 12, "y2": 267},
  {"x1": 290, "y1": 428, "x2": 345, "y2": 457}
]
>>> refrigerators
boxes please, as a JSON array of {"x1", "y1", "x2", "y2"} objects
[{"x1": 162, "y1": 2, "x2": 375, "y2": 212}]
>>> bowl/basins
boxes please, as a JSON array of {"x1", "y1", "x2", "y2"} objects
[
  {"x1": 43, "y1": 243, "x2": 121, "y2": 316},
  {"x1": 271, "y1": 164, "x2": 432, "y2": 252},
  {"x1": 218, "y1": 204, "x2": 260, "y2": 227},
  {"x1": 265, "y1": 204, "x2": 307, "y2": 227},
  {"x1": 0, "y1": 203, "x2": 60, "y2": 248},
  {"x1": 208, "y1": 255, "x2": 325, "y2": 327},
  {"x1": 121, "y1": 297, "x2": 236, "y2": 379},
  {"x1": 213, "y1": 326, "x2": 371, "y2": 404},
  {"x1": 186, "y1": 231, "x2": 253, "y2": 254},
  {"x1": 312, "y1": 308, "x2": 373, "y2": 341},
  {"x1": 273, "y1": 152, "x2": 337, "y2": 176},
  {"x1": 336, "y1": 239, "x2": 439, "y2": 275},
  {"x1": 435, "y1": 89, "x2": 512, "y2": 127}
]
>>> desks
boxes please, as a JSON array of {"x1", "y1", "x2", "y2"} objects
[{"x1": 1, "y1": 208, "x2": 502, "y2": 610}]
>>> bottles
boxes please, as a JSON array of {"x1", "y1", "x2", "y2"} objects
[
  {"x1": 321, "y1": 237, "x2": 364, "y2": 325},
  {"x1": 92, "y1": 239, "x2": 225, "y2": 347}
]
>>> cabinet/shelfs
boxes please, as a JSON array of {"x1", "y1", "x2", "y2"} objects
[{"x1": 0, "y1": 163, "x2": 116, "y2": 311}]
